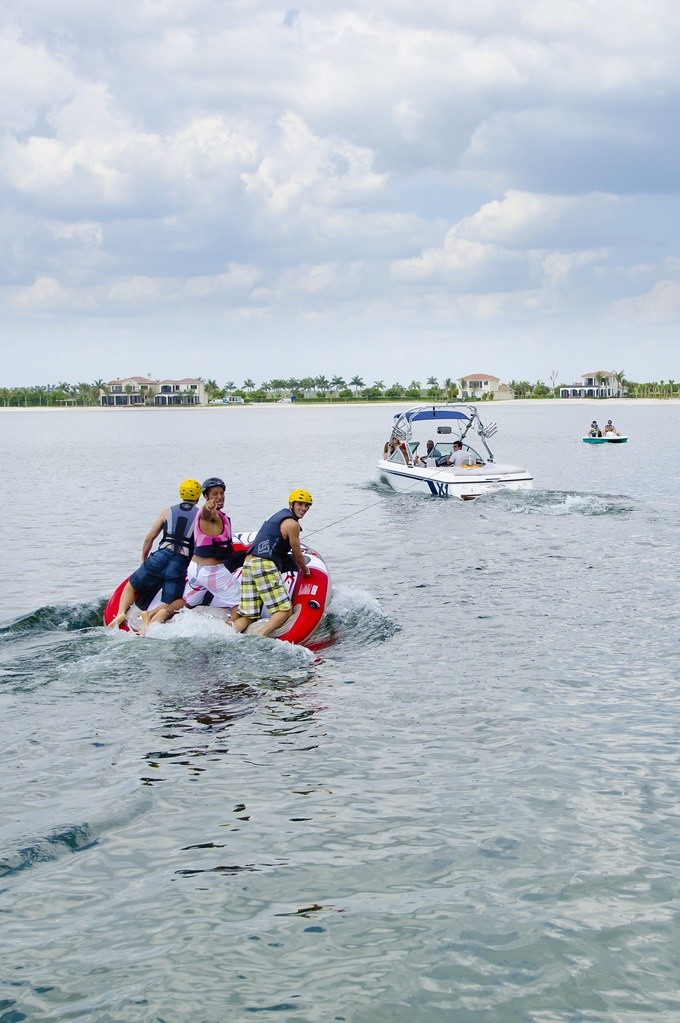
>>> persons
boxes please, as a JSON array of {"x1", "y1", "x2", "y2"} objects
[
  {"x1": 108, "y1": 480, "x2": 202, "y2": 634},
  {"x1": 426, "y1": 440, "x2": 441, "y2": 457},
  {"x1": 604, "y1": 420, "x2": 621, "y2": 436},
  {"x1": 383, "y1": 437, "x2": 400, "y2": 460},
  {"x1": 589, "y1": 421, "x2": 601, "y2": 437},
  {"x1": 447, "y1": 441, "x2": 469, "y2": 467},
  {"x1": 150, "y1": 477, "x2": 242, "y2": 623},
  {"x1": 232, "y1": 489, "x2": 313, "y2": 636}
]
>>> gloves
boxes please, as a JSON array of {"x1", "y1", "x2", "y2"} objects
[{"x1": 302, "y1": 567, "x2": 312, "y2": 578}]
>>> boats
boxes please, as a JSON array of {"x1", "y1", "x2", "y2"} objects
[
  {"x1": 582, "y1": 431, "x2": 629, "y2": 445},
  {"x1": 376, "y1": 404, "x2": 533, "y2": 501},
  {"x1": 102, "y1": 530, "x2": 335, "y2": 647}
]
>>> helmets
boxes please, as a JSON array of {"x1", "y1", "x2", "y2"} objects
[
  {"x1": 201, "y1": 478, "x2": 226, "y2": 494},
  {"x1": 179, "y1": 479, "x2": 201, "y2": 500},
  {"x1": 288, "y1": 489, "x2": 313, "y2": 504}
]
